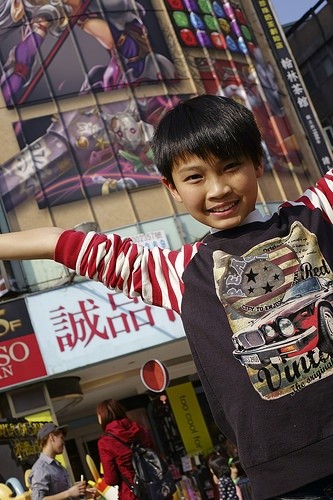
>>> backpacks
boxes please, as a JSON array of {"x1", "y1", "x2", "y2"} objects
[{"x1": 104, "y1": 433, "x2": 177, "y2": 500}]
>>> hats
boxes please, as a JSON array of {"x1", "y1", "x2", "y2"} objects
[{"x1": 37, "y1": 423, "x2": 68, "y2": 442}]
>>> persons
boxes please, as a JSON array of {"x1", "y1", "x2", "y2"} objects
[
  {"x1": 28, "y1": 422, "x2": 87, "y2": 499},
  {"x1": 90, "y1": 397, "x2": 174, "y2": 500},
  {"x1": 206, "y1": 440, "x2": 252, "y2": 500},
  {"x1": 0, "y1": 92, "x2": 333, "y2": 500}
]
